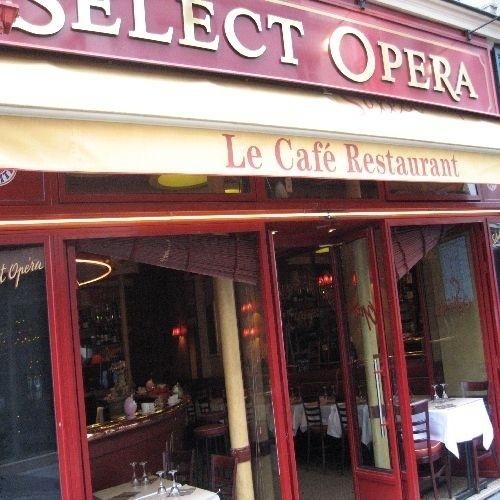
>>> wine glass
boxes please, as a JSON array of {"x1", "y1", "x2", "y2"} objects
[
  {"x1": 139, "y1": 461, "x2": 151, "y2": 486},
  {"x1": 167, "y1": 469, "x2": 181, "y2": 497},
  {"x1": 155, "y1": 469, "x2": 168, "y2": 496},
  {"x1": 431, "y1": 383, "x2": 450, "y2": 404},
  {"x1": 129, "y1": 461, "x2": 140, "y2": 487}
]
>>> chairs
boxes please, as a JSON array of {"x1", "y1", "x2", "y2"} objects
[
  {"x1": 300, "y1": 380, "x2": 499, "y2": 500},
  {"x1": 161, "y1": 388, "x2": 238, "y2": 500}
]
